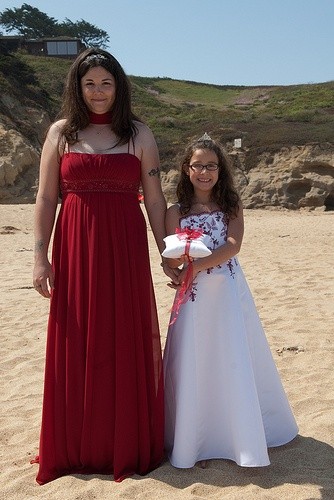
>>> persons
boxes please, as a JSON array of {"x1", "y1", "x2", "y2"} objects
[
  {"x1": 33, "y1": 47, "x2": 181, "y2": 485},
  {"x1": 161, "y1": 140, "x2": 300, "y2": 468}
]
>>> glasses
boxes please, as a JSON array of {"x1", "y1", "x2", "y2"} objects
[{"x1": 186, "y1": 163, "x2": 220, "y2": 172}]
